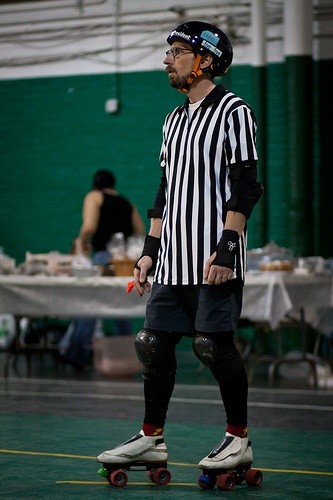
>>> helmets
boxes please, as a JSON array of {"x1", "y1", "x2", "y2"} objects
[{"x1": 167, "y1": 21, "x2": 233, "y2": 76}]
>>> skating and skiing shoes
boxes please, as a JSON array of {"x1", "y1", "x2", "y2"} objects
[
  {"x1": 96, "y1": 430, "x2": 171, "y2": 487},
  {"x1": 197, "y1": 432, "x2": 263, "y2": 491}
]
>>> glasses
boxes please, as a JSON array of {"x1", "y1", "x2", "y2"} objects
[{"x1": 165, "y1": 47, "x2": 197, "y2": 57}]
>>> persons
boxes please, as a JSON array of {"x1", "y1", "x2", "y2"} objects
[
  {"x1": 75, "y1": 169, "x2": 144, "y2": 371},
  {"x1": 97, "y1": 21, "x2": 262, "y2": 490}
]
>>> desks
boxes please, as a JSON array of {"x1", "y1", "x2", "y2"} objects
[{"x1": 0, "y1": 253, "x2": 333, "y2": 388}]
